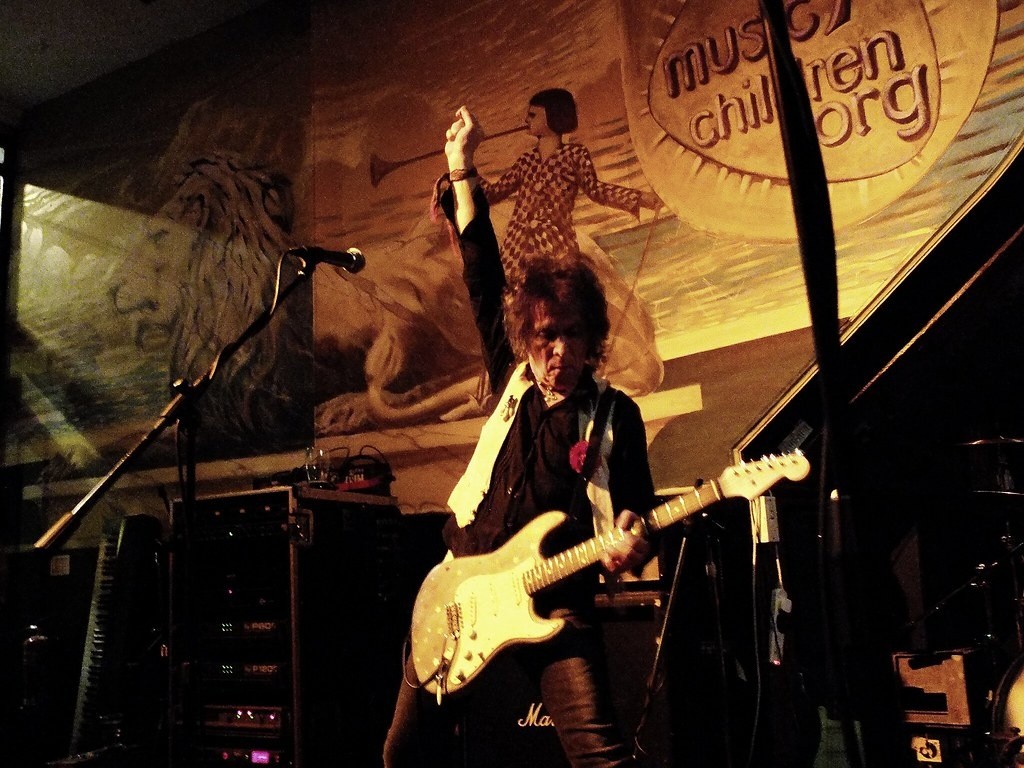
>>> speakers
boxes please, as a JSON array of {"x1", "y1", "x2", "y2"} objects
[{"x1": 466, "y1": 588, "x2": 719, "y2": 768}]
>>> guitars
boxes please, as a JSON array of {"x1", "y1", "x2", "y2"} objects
[{"x1": 411, "y1": 448, "x2": 811, "y2": 694}]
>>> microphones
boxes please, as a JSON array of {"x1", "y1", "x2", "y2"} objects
[{"x1": 288, "y1": 246, "x2": 366, "y2": 274}]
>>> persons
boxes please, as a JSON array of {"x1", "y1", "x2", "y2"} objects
[{"x1": 381, "y1": 255, "x2": 658, "y2": 768}]
